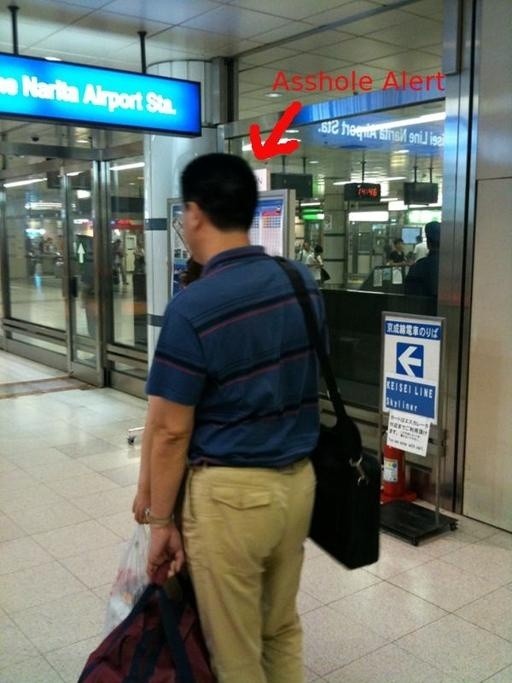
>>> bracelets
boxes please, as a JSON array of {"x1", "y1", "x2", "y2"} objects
[{"x1": 148, "y1": 515, "x2": 175, "y2": 526}]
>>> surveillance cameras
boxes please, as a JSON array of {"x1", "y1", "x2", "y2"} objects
[
  {"x1": 31, "y1": 136, "x2": 39, "y2": 141},
  {"x1": 353, "y1": 92, "x2": 358, "y2": 95}
]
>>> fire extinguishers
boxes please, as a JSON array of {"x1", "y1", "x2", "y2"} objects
[{"x1": 383, "y1": 430, "x2": 404, "y2": 498}]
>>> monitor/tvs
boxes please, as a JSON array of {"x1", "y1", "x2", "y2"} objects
[{"x1": 400, "y1": 226, "x2": 422, "y2": 245}]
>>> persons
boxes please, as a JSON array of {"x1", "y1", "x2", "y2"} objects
[
  {"x1": 387, "y1": 240, "x2": 406, "y2": 262},
  {"x1": 413, "y1": 235, "x2": 427, "y2": 262},
  {"x1": 297, "y1": 241, "x2": 313, "y2": 264},
  {"x1": 146, "y1": 152, "x2": 335, "y2": 682},
  {"x1": 132, "y1": 257, "x2": 219, "y2": 524},
  {"x1": 112, "y1": 239, "x2": 129, "y2": 285},
  {"x1": 23, "y1": 233, "x2": 64, "y2": 253},
  {"x1": 406, "y1": 221, "x2": 441, "y2": 314},
  {"x1": 306, "y1": 244, "x2": 328, "y2": 286},
  {"x1": 383, "y1": 238, "x2": 394, "y2": 265},
  {"x1": 407, "y1": 243, "x2": 419, "y2": 262}
]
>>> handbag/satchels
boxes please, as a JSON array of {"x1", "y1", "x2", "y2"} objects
[
  {"x1": 311, "y1": 420, "x2": 379, "y2": 569},
  {"x1": 321, "y1": 267, "x2": 331, "y2": 282},
  {"x1": 78, "y1": 565, "x2": 216, "y2": 683}
]
